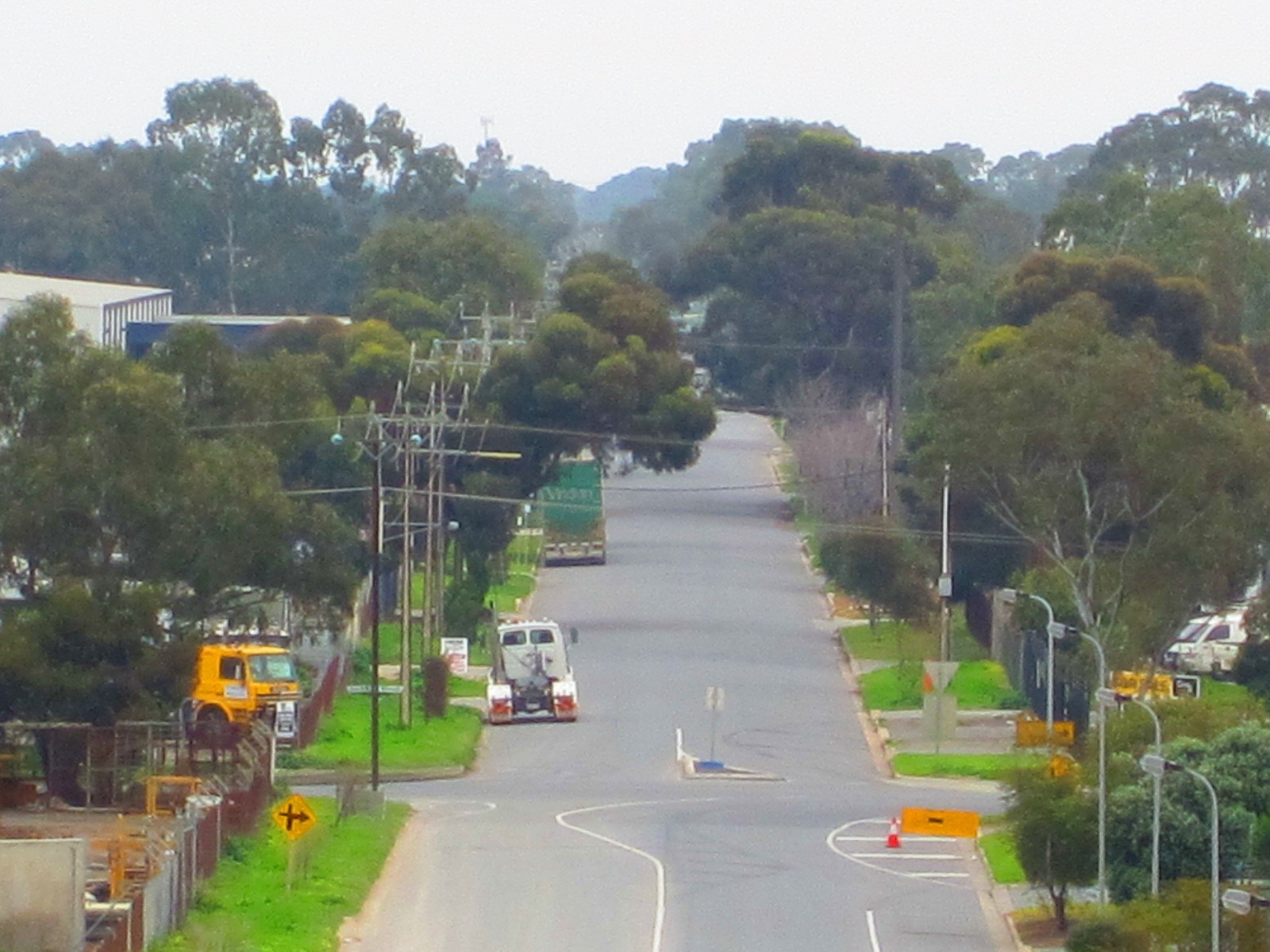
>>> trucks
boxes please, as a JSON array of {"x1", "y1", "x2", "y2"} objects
[
  {"x1": 537, "y1": 452, "x2": 609, "y2": 568},
  {"x1": 168, "y1": 639, "x2": 306, "y2": 751}
]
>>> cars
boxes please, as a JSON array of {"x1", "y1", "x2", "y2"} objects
[{"x1": 484, "y1": 616, "x2": 581, "y2": 725}]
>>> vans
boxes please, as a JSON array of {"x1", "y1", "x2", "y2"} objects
[{"x1": 1160, "y1": 610, "x2": 1270, "y2": 681}]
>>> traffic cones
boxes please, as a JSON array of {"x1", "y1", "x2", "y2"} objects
[{"x1": 884, "y1": 816, "x2": 902, "y2": 849}]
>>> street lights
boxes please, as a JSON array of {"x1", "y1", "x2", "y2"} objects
[
  {"x1": 1046, "y1": 621, "x2": 1108, "y2": 903},
  {"x1": 329, "y1": 431, "x2": 424, "y2": 791},
  {"x1": 1094, "y1": 684, "x2": 1163, "y2": 899},
  {"x1": 1138, "y1": 753, "x2": 1220, "y2": 952},
  {"x1": 997, "y1": 586, "x2": 1056, "y2": 871}
]
